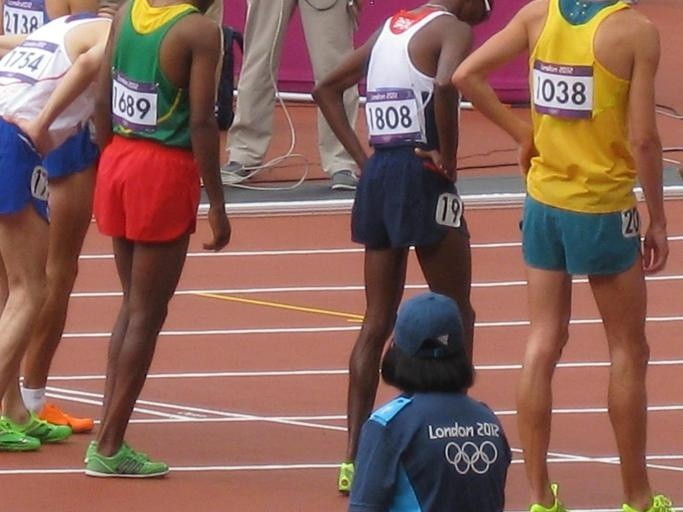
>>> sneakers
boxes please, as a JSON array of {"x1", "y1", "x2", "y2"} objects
[
  {"x1": 220, "y1": 160, "x2": 246, "y2": 182},
  {"x1": 85, "y1": 440, "x2": 148, "y2": 465},
  {"x1": 329, "y1": 167, "x2": 359, "y2": 192},
  {"x1": 620, "y1": 494, "x2": 677, "y2": 510},
  {"x1": 0, "y1": 416, "x2": 40, "y2": 453},
  {"x1": 525, "y1": 485, "x2": 569, "y2": 512},
  {"x1": 82, "y1": 443, "x2": 170, "y2": 479},
  {"x1": 338, "y1": 461, "x2": 354, "y2": 494},
  {"x1": 19, "y1": 418, "x2": 72, "y2": 444},
  {"x1": 35, "y1": 402, "x2": 92, "y2": 433}
]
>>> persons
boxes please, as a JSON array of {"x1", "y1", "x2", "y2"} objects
[
  {"x1": 20, "y1": 115, "x2": 102, "y2": 434},
  {"x1": 312, "y1": 1, "x2": 496, "y2": 495},
  {"x1": 0, "y1": 1, "x2": 128, "y2": 451},
  {"x1": 347, "y1": 291, "x2": 511, "y2": 511},
  {"x1": 82, "y1": 0, "x2": 230, "y2": 479},
  {"x1": 453, "y1": 1, "x2": 674, "y2": 511},
  {"x1": 221, "y1": 0, "x2": 361, "y2": 190},
  {"x1": 0, "y1": 0, "x2": 100, "y2": 61}
]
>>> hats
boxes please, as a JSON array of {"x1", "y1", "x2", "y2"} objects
[{"x1": 392, "y1": 293, "x2": 467, "y2": 360}]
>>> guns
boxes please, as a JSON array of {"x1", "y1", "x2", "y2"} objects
[{"x1": 347, "y1": 0, "x2": 361, "y2": 31}]
